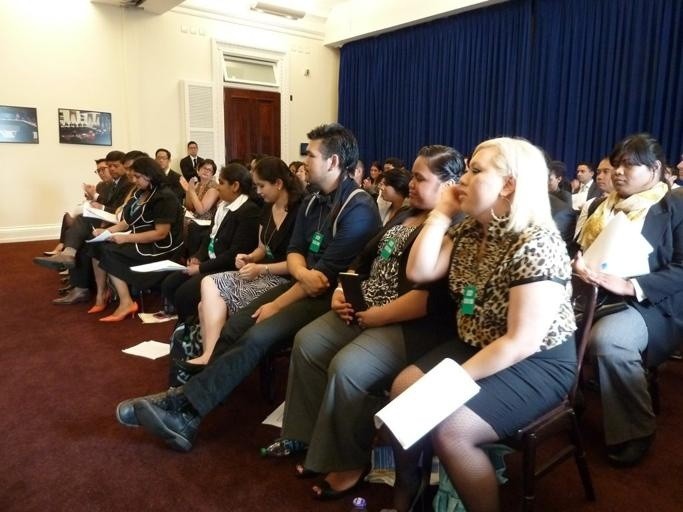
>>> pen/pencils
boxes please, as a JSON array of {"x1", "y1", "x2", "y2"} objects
[{"x1": 90, "y1": 223, "x2": 97, "y2": 233}]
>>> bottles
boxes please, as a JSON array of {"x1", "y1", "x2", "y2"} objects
[{"x1": 259, "y1": 437, "x2": 304, "y2": 458}]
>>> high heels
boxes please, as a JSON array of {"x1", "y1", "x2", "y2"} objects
[
  {"x1": 99, "y1": 302, "x2": 138, "y2": 322},
  {"x1": 88, "y1": 288, "x2": 112, "y2": 313}
]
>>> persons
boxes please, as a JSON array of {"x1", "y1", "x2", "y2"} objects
[
  {"x1": 390, "y1": 137, "x2": 581, "y2": 511},
  {"x1": 115, "y1": 124, "x2": 383, "y2": 454},
  {"x1": 34, "y1": 121, "x2": 680, "y2": 324},
  {"x1": 175, "y1": 156, "x2": 312, "y2": 374},
  {"x1": 281, "y1": 144, "x2": 467, "y2": 501},
  {"x1": 569, "y1": 133, "x2": 681, "y2": 470}
]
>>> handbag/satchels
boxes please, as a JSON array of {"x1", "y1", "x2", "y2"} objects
[{"x1": 170, "y1": 319, "x2": 205, "y2": 387}]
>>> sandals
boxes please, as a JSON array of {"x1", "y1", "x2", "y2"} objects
[
  {"x1": 295, "y1": 460, "x2": 324, "y2": 476},
  {"x1": 308, "y1": 468, "x2": 372, "y2": 501}
]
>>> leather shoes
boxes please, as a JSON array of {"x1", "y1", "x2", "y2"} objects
[
  {"x1": 34, "y1": 251, "x2": 91, "y2": 304},
  {"x1": 133, "y1": 399, "x2": 201, "y2": 452},
  {"x1": 172, "y1": 357, "x2": 209, "y2": 374},
  {"x1": 608, "y1": 433, "x2": 656, "y2": 467},
  {"x1": 116, "y1": 386, "x2": 192, "y2": 427}
]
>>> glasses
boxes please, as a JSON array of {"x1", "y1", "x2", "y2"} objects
[{"x1": 95, "y1": 166, "x2": 109, "y2": 173}]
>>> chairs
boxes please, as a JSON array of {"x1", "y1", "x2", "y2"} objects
[
  {"x1": 140, "y1": 217, "x2": 191, "y2": 312},
  {"x1": 511, "y1": 270, "x2": 600, "y2": 511}
]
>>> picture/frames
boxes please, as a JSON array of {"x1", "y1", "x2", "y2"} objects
[
  {"x1": 58, "y1": 108, "x2": 112, "y2": 147},
  {"x1": 0, "y1": 105, "x2": 39, "y2": 144}
]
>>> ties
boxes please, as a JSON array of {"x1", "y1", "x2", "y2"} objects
[{"x1": 194, "y1": 158, "x2": 196, "y2": 167}]
